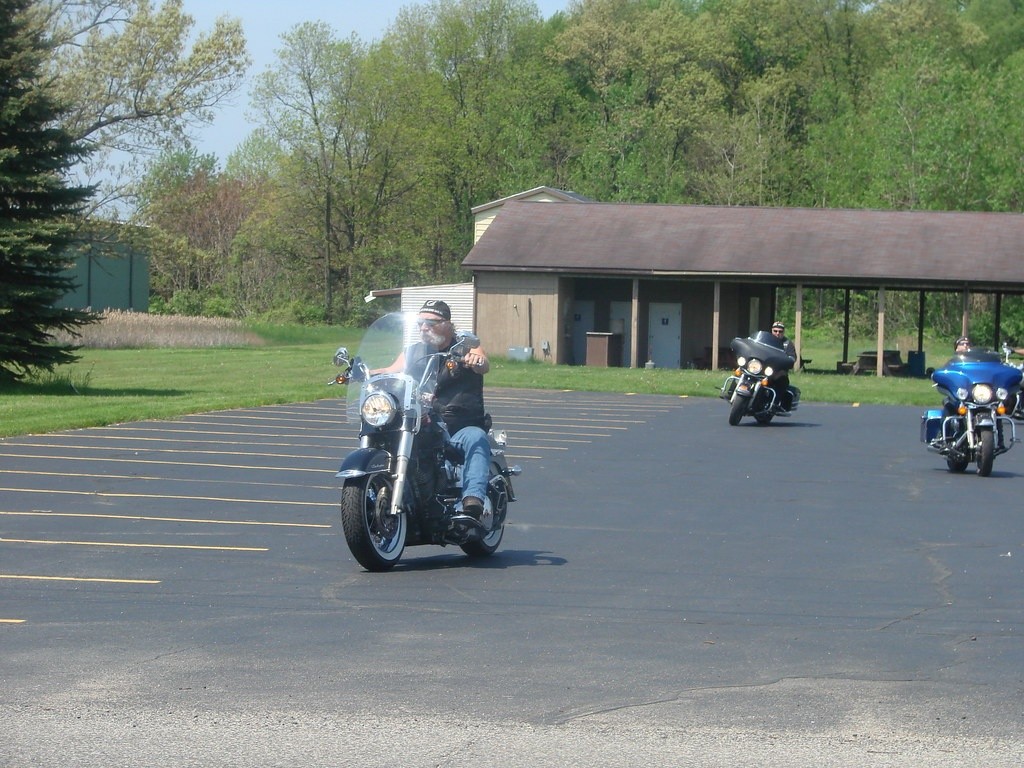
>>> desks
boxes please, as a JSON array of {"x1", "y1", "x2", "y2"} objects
[
  {"x1": 704, "y1": 346, "x2": 735, "y2": 367},
  {"x1": 850, "y1": 350, "x2": 900, "y2": 376},
  {"x1": 585, "y1": 332, "x2": 624, "y2": 368}
]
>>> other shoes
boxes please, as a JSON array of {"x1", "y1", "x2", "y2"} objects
[{"x1": 463, "y1": 497, "x2": 484, "y2": 520}]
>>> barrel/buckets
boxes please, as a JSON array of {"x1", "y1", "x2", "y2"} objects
[{"x1": 908, "y1": 351, "x2": 925, "y2": 376}]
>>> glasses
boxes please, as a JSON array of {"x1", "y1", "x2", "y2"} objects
[
  {"x1": 773, "y1": 330, "x2": 783, "y2": 333},
  {"x1": 418, "y1": 319, "x2": 447, "y2": 327},
  {"x1": 958, "y1": 343, "x2": 971, "y2": 346}
]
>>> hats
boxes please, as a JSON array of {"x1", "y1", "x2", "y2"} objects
[
  {"x1": 954, "y1": 337, "x2": 970, "y2": 350},
  {"x1": 772, "y1": 322, "x2": 785, "y2": 329},
  {"x1": 418, "y1": 300, "x2": 451, "y2": 321}
]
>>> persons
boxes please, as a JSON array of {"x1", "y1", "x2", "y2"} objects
[
  {"x1": 934, "y1": 336, "x2": 970, "y2": 448},
  {"x1": 1011, "y1": 349, "x2": 1024, "y2": 356},
  {"x1": 345, "y1": 300, "x2": 491, "y2": 519},
  {"x1": 719, "y1": 322, "x2": 797, "y2": 412}
]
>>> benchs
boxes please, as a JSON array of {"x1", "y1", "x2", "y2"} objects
[{"x1": 841, "y1": 361, "x2": 903, "y2": 370}]
